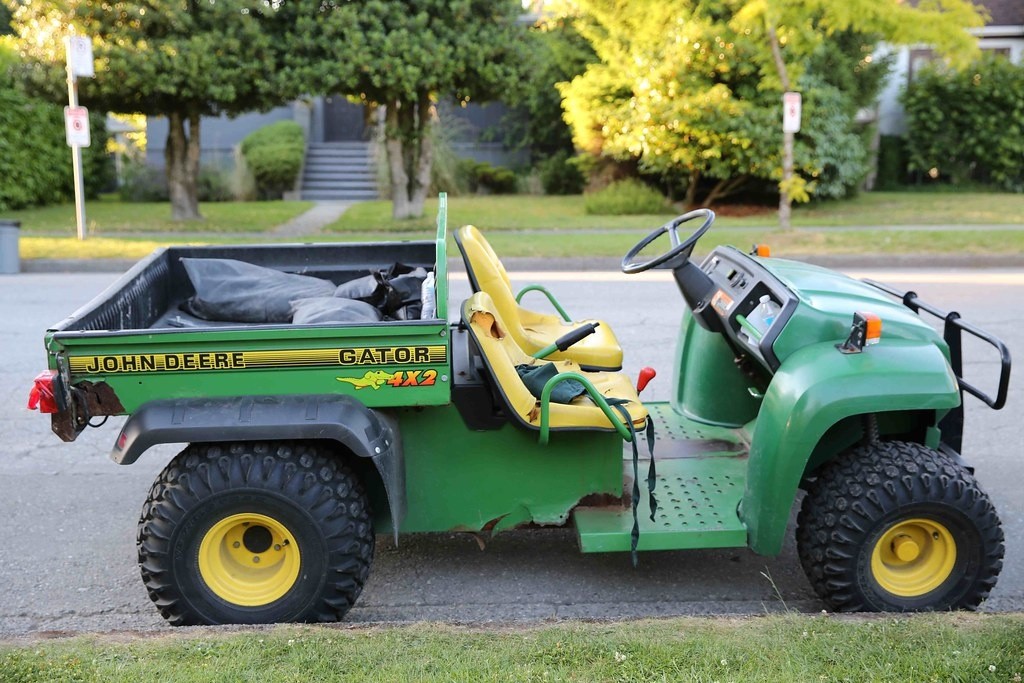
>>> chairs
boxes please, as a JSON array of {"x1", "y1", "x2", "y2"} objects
[
  {"x1": 460, "y1": 292, "x2": 647, "y2": 432},
  {"x1": 452, "y1": 224, "x2": 624, "y2": 373}
]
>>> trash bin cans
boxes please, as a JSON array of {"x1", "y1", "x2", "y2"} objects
[{"x1": 0, "y1": 220, "x2": 22, "y2": 274}]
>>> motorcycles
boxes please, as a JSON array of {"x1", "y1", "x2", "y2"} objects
[{"x1": 28, "y1": 192, "x2": 1012, "y2": 629}]
